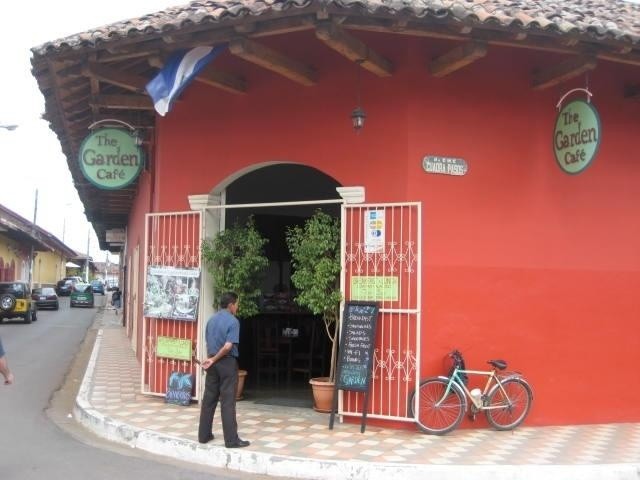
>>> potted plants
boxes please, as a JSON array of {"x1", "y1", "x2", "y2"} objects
[
  {"x1": 200, "y1": 213, "x2": 271, "y2": 400},
  {"x1": 285, "y1": 206, "x2": 344, "y2": 413}
]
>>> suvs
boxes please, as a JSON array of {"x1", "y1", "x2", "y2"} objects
[{"x1": 0, "y1": 283, "x2": 38, "y2": 325}]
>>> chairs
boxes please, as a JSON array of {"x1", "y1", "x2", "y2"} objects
[
  {"x1": 292, "y1": 314, "x2": 328, "y2": 386},
  {"x1": 257, "y1": 317, "x2": 292, "y2": 390}
]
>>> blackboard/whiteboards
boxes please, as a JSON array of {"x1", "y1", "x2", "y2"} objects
[
  {"x1": 165, "y1": 370, "x2": 193, "y2": 407},
  {"x1": 335, "y1": 300, "x2": 380, "y2": 391}
]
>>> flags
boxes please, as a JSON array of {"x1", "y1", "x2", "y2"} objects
[{"x1": 145, "y1": 43, "x2": 229, "y2": 117}]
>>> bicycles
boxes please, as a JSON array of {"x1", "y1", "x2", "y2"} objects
[{"x1": 405, "y1": 350, "x2": 535, "y2": 436}]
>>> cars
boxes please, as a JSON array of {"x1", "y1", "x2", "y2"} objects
[
  {"x1": 30, "y1": 288, "x2": 59, "y2": 310},
  {"x1": 71, "y1": 283, "x2": 94, "y2": 309},
  {"x1": 58, "y1": 279, "x2": 75, "y2": 296},
  {"x1": 91, "y1": 280, "x2": 106, "y2": 296}
]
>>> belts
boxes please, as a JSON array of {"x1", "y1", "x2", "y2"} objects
[{"x1": 221, "y1": 354, "x2": 235, "y2": 359}]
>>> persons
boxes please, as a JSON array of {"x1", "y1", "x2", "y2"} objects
[
  {"x1": 32, "y1": 289, "x2": 40, "y2": 321},
  {"x1": 198, "y1": 292, "x2": 251, "y2": 448},
  {"x1": 0, "y1": 339, "x2": 13, "y2": 385},
  {"x1": 112, "y1": 287, "x2": 122, "y2": 316}
]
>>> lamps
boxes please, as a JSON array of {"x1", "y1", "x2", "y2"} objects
[
  {"x1": 132, "y1": 88, "x2": 146, "y2": 152},
  {"x1": 351, "y1": 59, "x2": 367, "y2": 130}
]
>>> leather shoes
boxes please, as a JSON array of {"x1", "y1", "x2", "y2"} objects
[
  {"x1": 198, "y1": 433, "x2": 215, "y2": 444},
  {"x1": 226, "y1": 439, "x2": 250, "y2": 448}
]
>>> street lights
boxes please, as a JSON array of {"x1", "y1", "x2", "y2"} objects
[{"x1": 0, "y1": 122, "x2": 19, "y2": 135}]
